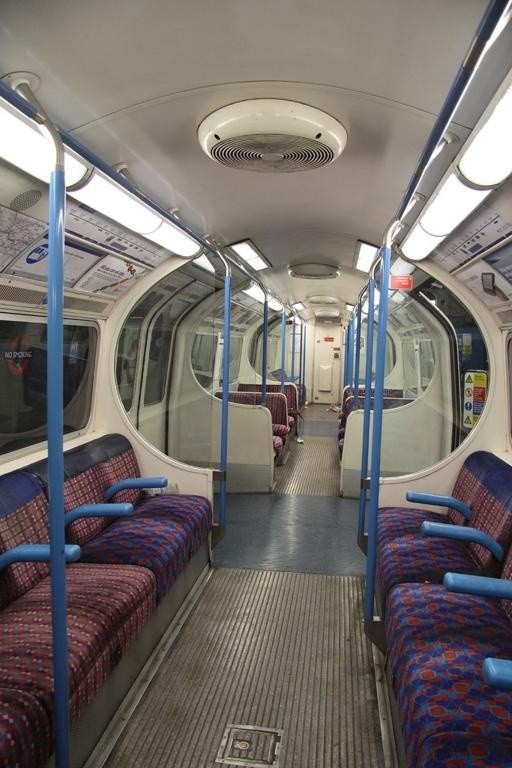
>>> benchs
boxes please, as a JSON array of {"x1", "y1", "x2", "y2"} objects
[
  {"x1": 17, "y1": 434, "x2": 210, "y2": 595},
  {"x1": 384, "y1": 544, "x2": 511, "y2": 766},
  {"x1": 2, "y1": 469, "x2": 158, "y2": 768},
  {"x1": 373, "y1": 452, "x2": 512, "y2": 618}
]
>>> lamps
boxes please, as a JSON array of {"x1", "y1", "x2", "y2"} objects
[
  {"x1": 2, "y1": 94, "x2": 300, "y2": 325},
  {"x1": 349, "y1": 67, "x2": 510, "y2": 334}
]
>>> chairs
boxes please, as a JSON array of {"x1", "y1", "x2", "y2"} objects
[
  {"x1": 210, "y1": 367, "x2": 306, "y2": 461},
  {"x1": 338, "y1": 385, "x2": 412, "y2": 459}
]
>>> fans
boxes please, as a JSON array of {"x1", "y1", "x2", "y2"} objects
[
  {"x1": 290, "y1": 264, "x2": 340, "y2": 276},
  {"x1": 202, "y1": 100, "x2": 348, "y2": 170}
]
